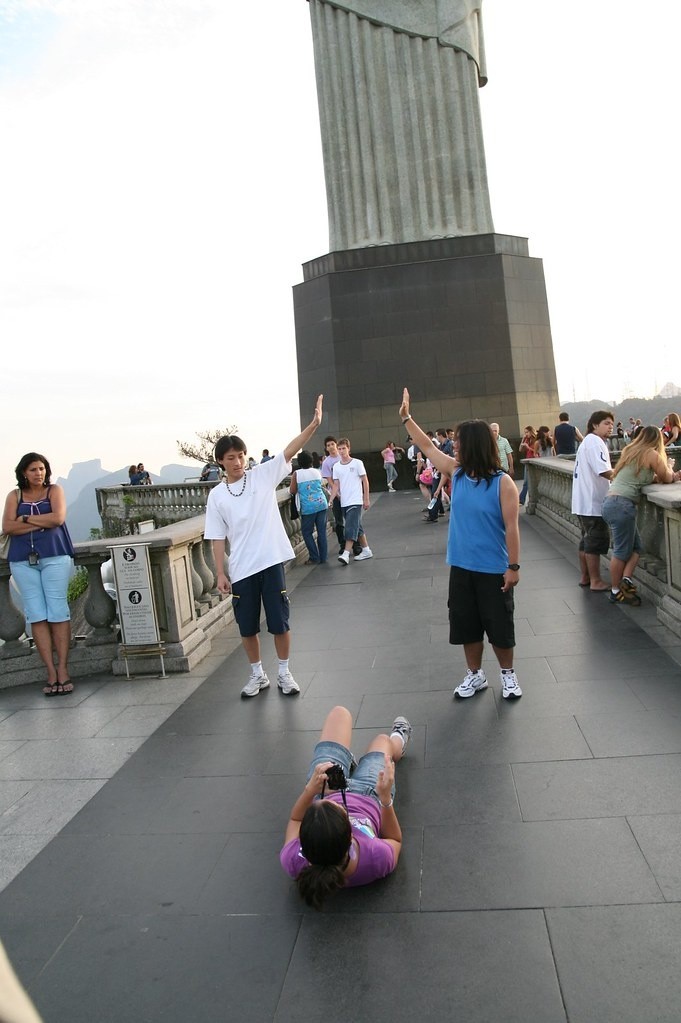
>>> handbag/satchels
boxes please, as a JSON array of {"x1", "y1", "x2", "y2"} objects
[
  {"x1": 290, "y1": 493, "x2": 299, "y2": 520},
  {"x1": 0, "y1": 488, "x2": 21, "y2": 560},
  {"x1": 199, "y1": 463, "x2": 210, "y2": 481},
  {"x1": 419, "y1": 468, "x2": 433, "y2": 485},
  {"x1": 394, "y1": 446, "x2": 402, "y2": 461}
]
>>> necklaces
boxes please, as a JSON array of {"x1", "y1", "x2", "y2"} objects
[{"x1": 226, "y1": 471, "x2": 247, "y2": 497}]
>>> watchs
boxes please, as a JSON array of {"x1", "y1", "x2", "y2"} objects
[
  {"x1": 506, "y1": 564, "x2": 520, "y2": 571},
  {"x1": 23, "y1": 514, "x2": 29, "y2": 523}
]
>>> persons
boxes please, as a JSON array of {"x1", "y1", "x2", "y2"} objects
[
  {"x1": 2, "y1": 452, "x2": 76, "y2": 697},
  {"x1": 406, "y1": 428, "x2": 455, "y2": 524},
  {"x1": 571, "y1": 411, "x2": 614, "y2": 593},
  {"x1": 601, "y1": 425, "x2": 681, "y2": 605},
  {"x1": 289, "y1": 450, "x2": 328, "y2": 565},
  {"x1": 617, "y1": 414, "x2": 681, "y2": 450},
  {"x1": 280, "y1": 706, "x2": 411, "y2": 911},
  {"x1": 204, "y1": 394, "x2": 324, "y2": 697},
  {"x1": 518, "y1": 425, "x2": 556, "y2": 505},
  {"x1": 201, "y1": 447, "x2": 330, "y2": 481},
  {"x1": 129, "y1": 463, "x2": 151, "y2": 485},
  {"x1": 553, "y1": 413, "x2": 583, "y2": 455},
  {"x1": 322, "y1": 435, "x2": 362, "y2": 556},
  {"x1": 381, "y1": 441, "x2": 405, "y2": 493},
  {"x1": 489, "y1": 423, "x2": 514, "y2": 477},
  {"x1": 400, "y1": 388, "x2": 522, "y2": 699},
  {"x1": 329, "y1": 438, "x2": 373, "y2": 565}
]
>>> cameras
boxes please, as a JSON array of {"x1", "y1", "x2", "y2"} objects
[
  {"x1": 325, "y1": 765, "x2": 348, "y2": 790},
  {"x1": 28, "y1": 552, "x2": 40, "y2": 566}
]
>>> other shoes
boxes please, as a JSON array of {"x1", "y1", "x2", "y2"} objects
[
  {"x1": 438, "y1": 513, "x2": 445, "y2": 517},
  {"x1": 423, "y1": 514, "x2": 438, "y2": 522},
  {"x1": 339, "y1": 548, "x2": 344, "y2": 554},
  {"x1": 353, "y1": 544, "x2": 361, "y2": 554},
  {"x1": 304, "y1": 558, "x2": 321, "y2": 564},
  {"x1": 421, "y1": 508, "x2": 428, "y2": 512}
]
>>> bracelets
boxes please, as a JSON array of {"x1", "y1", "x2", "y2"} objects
[
  {"x1": 402, "y1": 414, "x2": 411, "y2": 424},
  {"x1": 416, "y1": 472, "x2": 420, "y2": 474},
  {"x1": 379, "y1": 799, "x2": 393, "y2": 808}
]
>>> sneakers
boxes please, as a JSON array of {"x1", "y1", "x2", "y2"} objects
[
  {"x1": 337, "y1": 553, "x2": 349, "y2": 565},
  {"x1": 500, "y1": 668, "x2": 522, "y2": 699},
  {"x1": 608, "y1": 588, "x2": 642, "y2": 606},
  {"x1": 387, "y1": 483, "x2": 393, "y2": 489},
  {"x1": 453, "y1": 668, "x2": 487, "y2": 698},
  {"x1": 354, "y1": 549, "x2": 373, "y2": 560},
  {"x1": 389, "y1": 489, "x2": 397, "y2": 492},
  {"x1": 391, "y1": 714, "x2": 413, "y2": 757},
  {"x1": 620, "y1": 578, "x2": 638, "y2": 594},
  {"x1": 240, "y1": 672, "x2": 270, "y2": 698},
  {"x1": 277, "y1": 671, "x2": 300, "y2": 696}
]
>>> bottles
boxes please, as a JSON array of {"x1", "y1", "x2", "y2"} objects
[{"x1": 427, "y1": 497, "x2": 437, "y2": 509}]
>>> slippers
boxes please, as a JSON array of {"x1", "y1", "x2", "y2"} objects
[
  {"x1": 590, "y1": 585, "x2": 612, "y2": 592},
  {"x1": 43, "y1": 679, "x2": 73, "y2": 696},
  {"x1": 579, "y1": 579, "x2": 590, "y2": 586}
]
